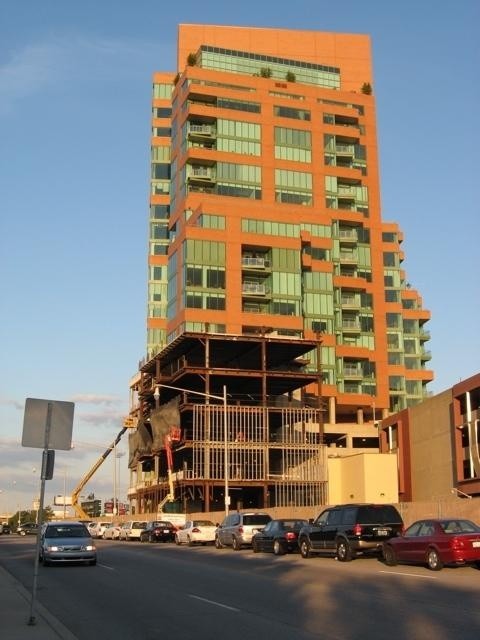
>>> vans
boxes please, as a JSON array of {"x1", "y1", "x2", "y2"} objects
[
  {"x1": 118, "y1": 520, "x2": 149, "y2": 541},
  {"x1": 215, "y1": 510, "x2": 274, "y2": 550}
]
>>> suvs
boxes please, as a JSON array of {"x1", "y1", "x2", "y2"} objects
[
  {"x1": 85, "y1": 522, "x2": 96, "y2": 533},
  {"x1": 298, "y1": 502, "x2": 404, "y2": 562}
]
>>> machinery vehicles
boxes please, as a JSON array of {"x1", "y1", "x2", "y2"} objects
[
  {"x1": 157, "y1": 425, "x2": 183, "y2": 512},
  {"x1": 72, "y1": 414, "x2": 142, "y2": 519}
]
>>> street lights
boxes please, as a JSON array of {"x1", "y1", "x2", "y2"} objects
[
  {"x1": 68, "y1": 439, "x2": 118, "y2": 516},
  {"x1": 153, "y1": 381, "x2": 231, "y2": 519},
  {"x1": 0, "y1": 490, "x2": 9, "y2": 525},
  {"x1": 11, "y1": 480, "x2": 21, "y2": 524},
  {"x1": 32, "y1": 466, "x2": 66, "y2": 518},
  {"x1": 450, "y1": 488, "x2": 472, "y2": 499}
]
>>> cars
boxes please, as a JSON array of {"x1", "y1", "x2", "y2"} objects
[
  {"x1": 253, "y1": 518, "x2": 308, "y2": 555},
  {"x1": 16, "y1": 521, "x2": 40, "y2": 536},
  {"x1": 140, "y1": 519, "x2": 177, "y2": 543},
  {"x1": 38, "y1": 521, "x2": 97, "y2": 567},
  {"x1": 174, "y1": 519, "x2": 219, "y2": 546},
  {"x1": 90, "y1": 521, "x2": 111, "y2": 539},
  {"x1": 381, "y1": 518, "x2": 480, "y2": 571},
  {"x1": 102, "y1": 522, "x2": 125, "y2": 540},
  {"x1": 2, "y1": 524, "x2": 13, "y2": 535}
]
